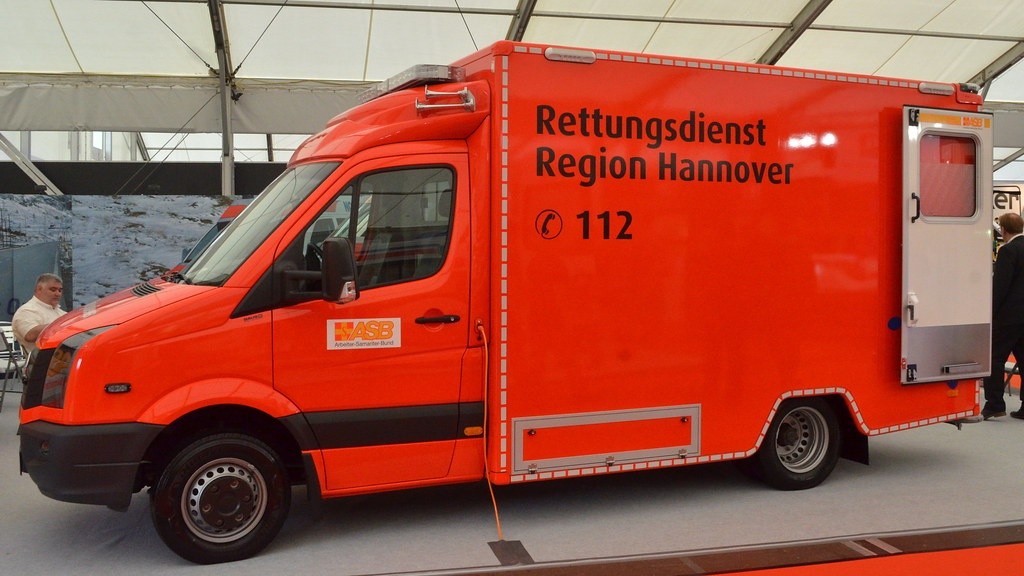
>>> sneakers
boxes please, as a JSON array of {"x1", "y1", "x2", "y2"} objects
[
  {"x1": 982, "y1": 399, "x2": 1007, "y2": 418},
  {"x1": 1011, "y1": 402, "x2": 1024, "y2": 418}
]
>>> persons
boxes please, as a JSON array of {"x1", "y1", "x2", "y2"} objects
[
  {"x1": 980, "y1": 213, "x2": 1023, "y2": 419},
  {"x1": 10, "y1": 273, "x2": 68, "y2": 364}
]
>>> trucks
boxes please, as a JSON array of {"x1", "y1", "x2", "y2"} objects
[{"x1": 15, "y1": 41, "x2": 997, "y2": 561}]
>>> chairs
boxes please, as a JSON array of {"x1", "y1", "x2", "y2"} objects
[
  {"x1": 414, "y1": 233, "x2": 446, "y2": 283},
  {"x1": 0, "y1": 329, "x2": 25, "y2": 413},
  {"x1": 1005, "y1": 359, "x2": 1019, "y2": 396},
  {"x1": 0, "y1": 321, "x2": 25, "y2": 389},
  {"x1": 358, "y1": 232, "x2": 402, "y2": 287}
]
